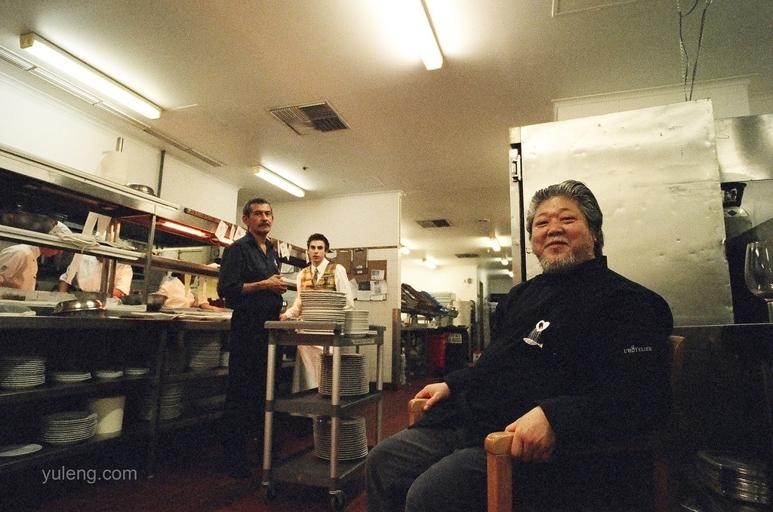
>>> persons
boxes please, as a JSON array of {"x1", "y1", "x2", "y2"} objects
[
  {"x1": 0, "y1": 241, "x2": 63, "y2": 290},
  {"x1": 365, "y1": 179, "x2": 675, "y2": 512},
  {"x1": 57, "y1": 242, "x2": 134, "y2": 298},
  {"x1": 280, "y1": 233, "x2": 354, "y2": 443},
  {"x1": 197, "y1": 257, "x2": 231, "y2": 311},
  {"x1": 156, "y1": 269, "x2": 196, "y2": 309},
  {"x1": 215, "y1": 199, "x2": 289, "y2": 480}
]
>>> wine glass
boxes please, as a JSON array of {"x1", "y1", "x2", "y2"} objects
[{"x1": 743, "y1": 240, "x2": 773, "y2": 323}]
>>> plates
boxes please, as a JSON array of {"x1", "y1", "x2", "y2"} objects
[
  {"x1": 105, "y1": 302, "x2": 233, "y2": 321},
  {"x1": 317, "y1": 352, "x2": 370, "y2": 397},
  {"x1": 0, "y1": 411, "x2": 97, "y2": 458},
  {"x1": 138, "y1": 384, "x2": 183, "y2": 420},
  {"x1": 312, "y1": 416, "x2": 368, "y2": 462},
  {"x1": 163, "y1": 330, "x2": 222, "y2": 374},
  {"x1": 0, "y1": 356, "x2": 150, "y2": 389},
  {"x1": 299, "y1": 289, "x2": 370, "y2": 338}
]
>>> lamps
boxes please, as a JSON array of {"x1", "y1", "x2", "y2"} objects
[
  {"x1": 401, "y1": 2, "x2": 444, "y2": 76},
  {"x1": 17, "y1": 32, "x2": 182, "y2": 124}
]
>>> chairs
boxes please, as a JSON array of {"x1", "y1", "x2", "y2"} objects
[{"x1": 408, "y1": 332, "x2": 686, "y2": 511}]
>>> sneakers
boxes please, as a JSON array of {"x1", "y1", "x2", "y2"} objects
[{"x1": 220, "y1": 435, "x2": 252, "y2": 481}]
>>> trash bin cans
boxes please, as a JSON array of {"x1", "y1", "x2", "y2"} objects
[{"x1": 425, "y1": 324, "x2": 468, "y2": 376}]
